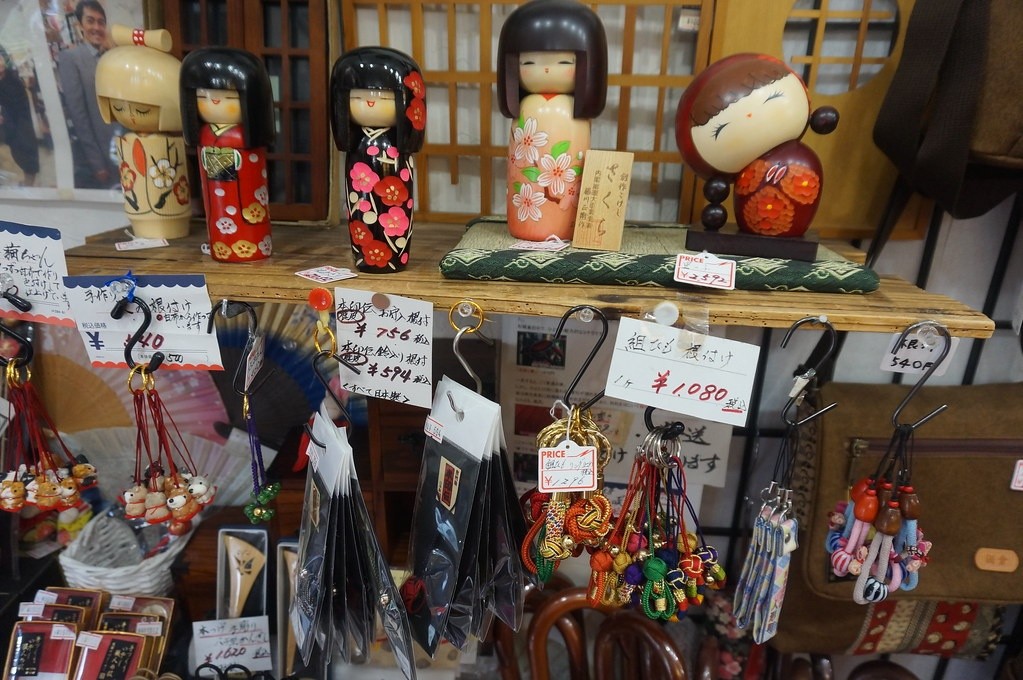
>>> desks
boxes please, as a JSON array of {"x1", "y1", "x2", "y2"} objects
[{"x1": 60, "y1": 217, "x2": 994, "y2": 680}]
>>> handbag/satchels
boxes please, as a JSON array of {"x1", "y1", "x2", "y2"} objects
[{"x1": 758, "y1": 384, "x2": 1023, "y2": 661}]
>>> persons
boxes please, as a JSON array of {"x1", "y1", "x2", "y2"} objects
[
  {"x1": 674, "y1": 52, "x2": 840, "y2": 239},
  {"x1": 94, "y1": 23, "x2": 193, "y2": 216},
  {"x1": 329, "y1": 46, "x2": 426, "y2": 273},
  {"x1": 179, "y1": 47, "x2": 277, "y2": 263},
  {"x1": 57, "y1": 0, "x2": 123, "y2": 189},
  {"x1": 0, "y1": 45, "x2": 40, "y2": 187},
  {"x1": 496, "y1": 0, "x2": 609, "y2": 242}
]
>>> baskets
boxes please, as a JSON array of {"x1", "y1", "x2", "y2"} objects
[{"x1": 59, "y1": 499, "x2": 209, "y2": 610}]
